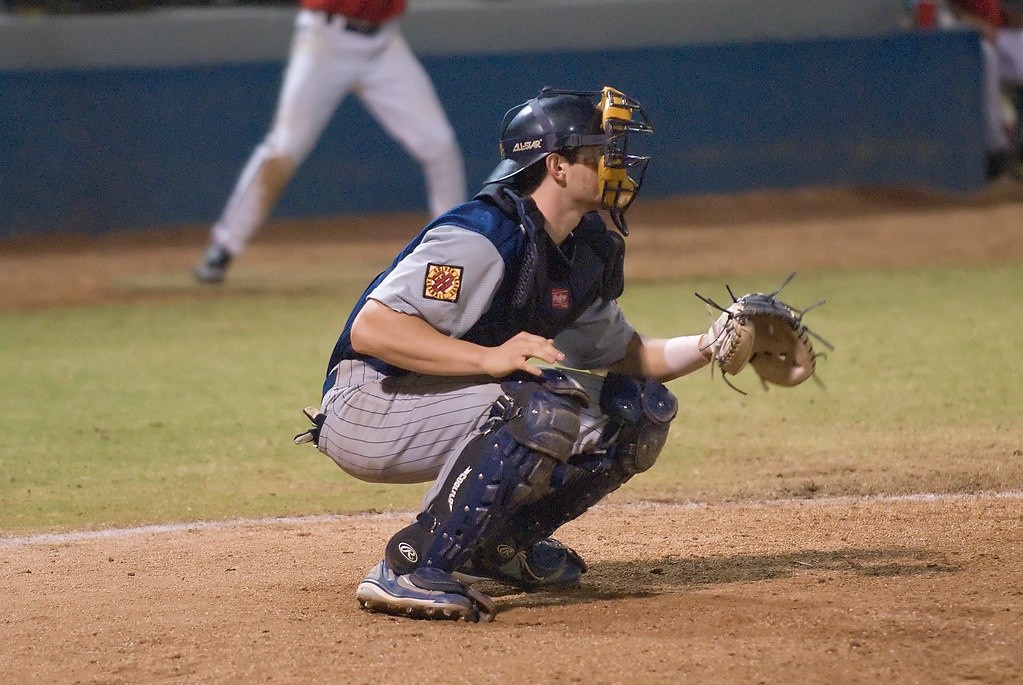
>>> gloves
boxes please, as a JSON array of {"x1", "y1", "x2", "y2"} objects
[{"x1": 294, "y1": 406, "x2": 327, "y2": 449}]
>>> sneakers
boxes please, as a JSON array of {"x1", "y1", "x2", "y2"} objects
[
  {"x1": 355, "y1": 559, "x2": 476, "y2": 621},
  {"x1": 451, "y1": 557, "x2": 581, "y2": 592}
]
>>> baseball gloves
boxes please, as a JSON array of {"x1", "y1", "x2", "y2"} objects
[{"x1": 693, "y1": 270, "x2": 835, "y2": 396}]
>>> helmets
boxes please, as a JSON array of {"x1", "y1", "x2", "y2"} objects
[{"x1": 482, "y1": 93, "x2": 601, "y2": 185}]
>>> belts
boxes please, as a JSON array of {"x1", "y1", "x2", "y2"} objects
[
  {"x1": 322, "y1": 359, "x2": 403, "y2": 401},
  {"x1": 325, "y1": 14, "x2": 380, "y2": 35}
]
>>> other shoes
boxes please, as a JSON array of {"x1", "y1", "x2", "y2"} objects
[{"x1": 196, "y1": 245, "x2": 232, "y2": 281}]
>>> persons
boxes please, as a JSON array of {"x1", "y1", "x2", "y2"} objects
[
  {"x1": 919, "y1": 0, "x2": 1023, "y2": 179},
  {"x1": 197, "y1": 0, "x2": 467, "y2": 283},
  {"x1": 314, "y1": 86, "x2": 816, "y2": 623}
]
